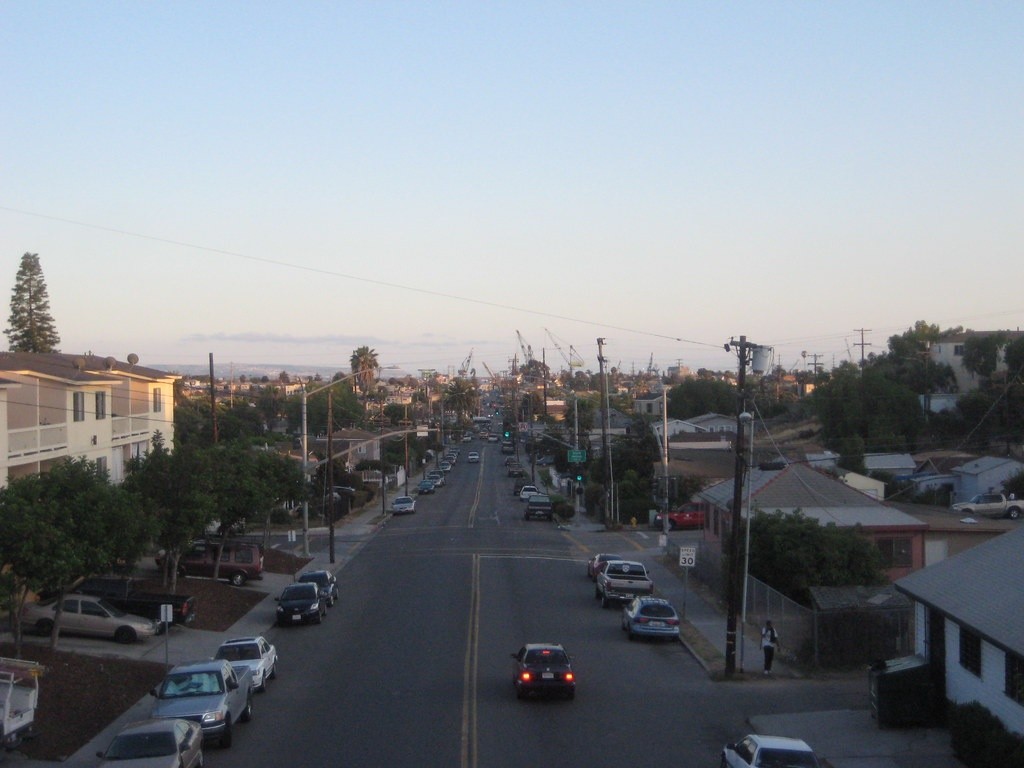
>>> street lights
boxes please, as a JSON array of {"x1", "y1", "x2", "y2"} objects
[
  {"x1": 603, "y1": 357, "x2": 617, "y2": 524},
  {"x1": 661, "y1": 384, "x2": 672, "y2": 536},
  {"x1": 299, "y1": 365, "x2": 401, "y2": 559},
  {"x1": 738, "y1": 411, "x2": 760, "y2": 671}
]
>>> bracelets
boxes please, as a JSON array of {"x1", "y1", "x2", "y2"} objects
[{"x1": 776, "y1": 646, "x2": 780, "y2": 648}]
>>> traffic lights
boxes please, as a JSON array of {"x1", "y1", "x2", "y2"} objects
[
  {"x1": 576, "y1": 474, "x2": 586, "y2": 483},
  {"x1": 502, "y1": 421, "x2": 513, "y2": 442},
  {"x1": 496, "y1": 408, "x2": 499, "y2": 415}
]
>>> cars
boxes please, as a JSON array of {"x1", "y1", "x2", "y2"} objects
[
  {"x1": 298, "y1": 569, "x2": 344, "y2": 608},
  {"x1": 622, "y1": 596, "x2": 681, "y2": 638},
  {"x1": 419, "y1": 412, "x2": 540, "y2": 498},
  {"x1": 210, "y1": 636, "x2": 282, "y2": 691},
  {"x1": 468, "y1": 451, "x2": 482, "y2": 464},
  {"x1": 588, "y1": 554, "x2": 625, "y2": 581},
  {"x1": 520, "y1": 486, "x2": 541, "y2": 502},
  {"x1": 720, "y1": 734, "x2": 819, "y2": 768},
  {"x1": 94, "y1": 718, "x2": 205, "y2": 767},
  {"x1": 391, "y1": 496, "x2": 417, "y2": 515},
  {"x1": 22, "y1": 593, "x2": 156, "y2": 644}
]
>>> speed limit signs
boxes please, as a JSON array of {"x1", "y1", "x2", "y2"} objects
[{"x1": 679, "y1": 547, "x2": 697, "y2": 568}]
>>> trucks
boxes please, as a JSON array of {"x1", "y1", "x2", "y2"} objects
[{"x1": 0, "y1": 657, "x2": 47, "y2": 751}]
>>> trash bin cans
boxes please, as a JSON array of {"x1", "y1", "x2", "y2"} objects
[{"x1": 869, "y1": 653, "x2": 932, "y2": 729}]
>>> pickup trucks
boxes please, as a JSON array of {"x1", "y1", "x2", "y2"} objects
[
  {"x1": 77, "y1": 578, "x2": 197, "y2": 635},
  {"x1": 950, "y1": 492, "x2": 1024, "y2": 520},
  {"x1": 148, "y1": 658, "x2": 257, "y2": 747},
  {"x1": 596, "y1": 559, "x2": 654, "y2": 607}
]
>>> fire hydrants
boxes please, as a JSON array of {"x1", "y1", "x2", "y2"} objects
[{"x1": 630, "y1": 517, "x2": 638, "y2": 530}]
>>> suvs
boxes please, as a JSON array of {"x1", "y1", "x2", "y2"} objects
[
  {"x1": 274, "y1": 582, "x2": 330, "y2": 626},
  {"x1": 524, "y1": 495, "x2": 555, "y2": 521},
  {"x1": 155, "y1": 539, "x2": 271, "y2": 585},
  {"x1": 654, "y1": 501, "x2": 707, "y2": 532},
  {"x1": 510, "y1": 642, "x2": 579, "y2": 702}
]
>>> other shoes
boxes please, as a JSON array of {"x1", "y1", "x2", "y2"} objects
[{"x1": 764, "y1": 670, "x2": 772, "y2": 674}]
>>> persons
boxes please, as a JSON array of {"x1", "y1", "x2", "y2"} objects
[
  {"x1": 760, "y1": 619, "x2": 780, "y2": 675},
  {"x1": 1007, "y1": 489, "x2": 1015, "y2": 501}
]
